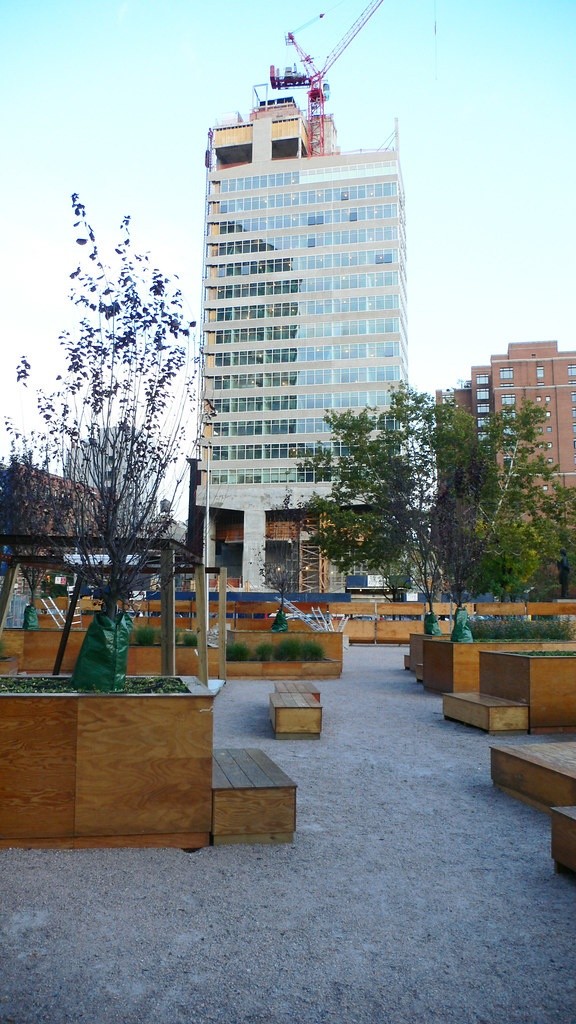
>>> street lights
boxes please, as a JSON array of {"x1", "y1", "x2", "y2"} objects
[{"x1": 196, "y1": 440, "x2": 210, "y2": 633}]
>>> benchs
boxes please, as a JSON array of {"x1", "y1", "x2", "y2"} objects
[
  {"x1": 211, "y1": 748, "x2": 298, "y2": 845},
  {"x1": 488, "y1": 742, "x2": 576, "y2": 875},
  {"x1": 415, "y1": 663, "x2": 423, "y2": 683},
  {"x1": 441, "y1": 692, "x2": 529, "y2": 735},
  {"x1": 404, "y1": 655, "x2": 410, "y2": 669}
]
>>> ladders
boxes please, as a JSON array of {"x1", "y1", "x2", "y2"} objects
[
  {"x1": 324, "y1": 614, "x2": 334, "y2": 631},
  {"x1": 274, "y1": 596, "x2": 324, "y2": 631},
  {"x1": 336, "y1": 616, "x2": 349, "y2": 632},
  {"x1": 312, "y1": 606, "x2": 330, "y2": 631},
  {"x1": 67, "y1": 582, "x2": 82, "y2": 629},
  {"x1": 41, "y1": 596, "x2": 66, "y2": 628}
]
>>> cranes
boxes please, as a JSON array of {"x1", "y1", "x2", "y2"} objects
[{"x1": 270, "y1": 1, "x2": 385, "y2": 159}]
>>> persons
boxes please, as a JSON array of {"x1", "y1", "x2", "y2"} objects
[{"x1": 558, "y1": 548, "x2": 571, "y2": 598}]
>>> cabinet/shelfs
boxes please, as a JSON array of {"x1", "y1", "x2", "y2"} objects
[{"x1": 268, "y1": 680, "x2": 323, "y2": 740}]
